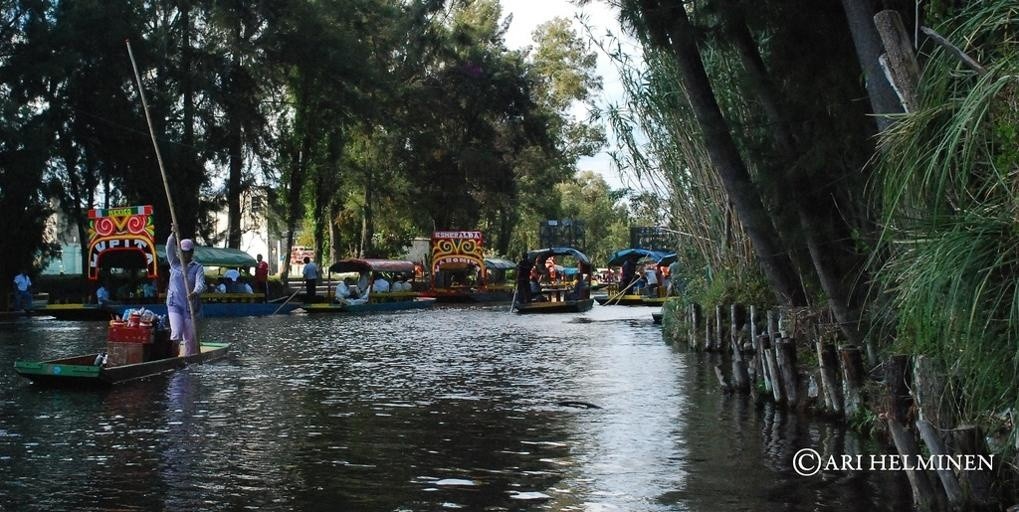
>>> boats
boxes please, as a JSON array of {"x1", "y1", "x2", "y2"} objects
[
  {"x1": 300, "y1": 255, "x2": 438, "y2": 314},
  {"x1": 511, "y1": 245, "x2": 598, "y2": 314},
  {"x1": 10, "y1": 336, "x2": 235, "y2": 390},
  {"x1": 592, "y1": 246, "x2": 682, "y2": 307},
  {"x1": 426, "y1": 230, "x2": 520, "y2": 304},
  {"x1": 80, "y1": 205, "x2": 304, "y2": 318}
]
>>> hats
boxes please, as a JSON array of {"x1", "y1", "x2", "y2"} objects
[{"x1": 180, "y1": 239, "x2": 195, "y2": 252}]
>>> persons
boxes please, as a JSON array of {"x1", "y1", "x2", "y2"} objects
[
  {"x1": 165, "y1": 223, "x2": 206, "y2": 355},
  {"x1": 208, "y1": 254, "x2": 268, "y2": 304},
  {"x1": 12, "y1": 268, "x2": 33, "y2": 310},
  {"x1": 619, "y1": 254, "x2": 681, "y2": 298},
  {"x1": 516, "y1": 251, "x2": 588, "y2": 303},
  {"x1": 302, "y1": 257, "x2": 318, "y2": 303},
  {"x1": 96, "y1": 275, "x2": 157, "y2": 305},
  {"x1": 334, "y1": 265, "x2": 415, "y2": 306}
]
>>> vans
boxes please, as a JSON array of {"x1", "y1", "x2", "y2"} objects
[{"x1": 291, "y1": 245, "x2": 315, "y2": 264}]
